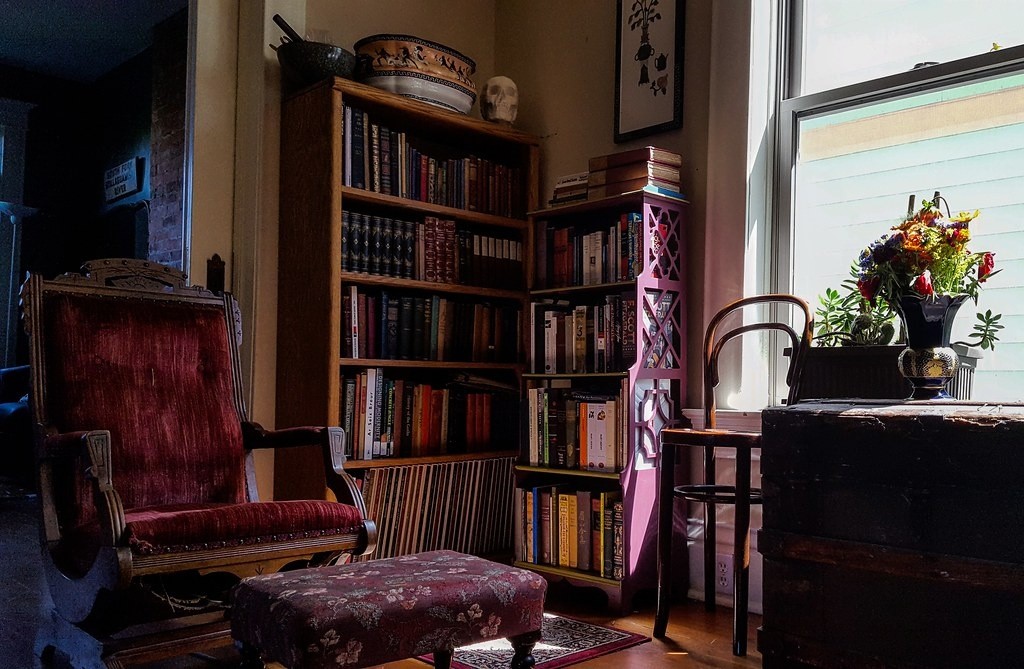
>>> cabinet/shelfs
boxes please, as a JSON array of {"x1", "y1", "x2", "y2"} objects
[
  {"x1": 276, "y1": 75, "x2": 542, "y2": 568},
  {"x1": 513, "y1": 194, "x2": 690, "y2": 619},
  {"x1": 757, "y1": 400, "x2": 1024, "y2": 669}
]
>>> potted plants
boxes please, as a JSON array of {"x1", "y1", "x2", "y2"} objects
[{"x1": 783, "y1": 282, "x2": 1004, "y2": 400}]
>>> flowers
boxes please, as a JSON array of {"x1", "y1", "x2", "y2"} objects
[{"x1": 855, "y1": 200, "x2": 994, "y2": 305}]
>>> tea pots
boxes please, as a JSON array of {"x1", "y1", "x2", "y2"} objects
[{"x1": 654, "y1": 53, "x2": 669, "y2": 71}]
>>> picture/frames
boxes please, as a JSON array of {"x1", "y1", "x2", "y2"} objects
[{"x1": 614, "y1": 1, "x2": 682, "y2": 143}]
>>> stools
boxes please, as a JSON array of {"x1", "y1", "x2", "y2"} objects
[
  {"x1": 228, "y1": 550, "x2": 547, "y2": 669},
  {"x1": 654, "y1": 291, "x2": 811, "y2": 654}
]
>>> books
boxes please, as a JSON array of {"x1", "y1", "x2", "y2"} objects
[{"x1": 339, "y1": 106, "x2": 682, "y2": 585}]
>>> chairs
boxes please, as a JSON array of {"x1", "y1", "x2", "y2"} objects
[{"x1": 18, "y1": 259, "x2": 377, "y2": 669}]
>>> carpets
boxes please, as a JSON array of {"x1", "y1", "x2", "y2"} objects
[{"x1": 416, "y1": 613, "x2": 650, "y2": 669}]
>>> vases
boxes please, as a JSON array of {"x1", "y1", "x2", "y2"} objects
[{"x1": 891, "y1": 293, "x2": 968, "y2": 401}]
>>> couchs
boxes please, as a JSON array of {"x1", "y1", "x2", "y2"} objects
[{"x1": 1, "y1": 364, "x2": 33, "y2": 492}]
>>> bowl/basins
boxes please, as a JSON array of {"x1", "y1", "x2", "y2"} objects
[
  {"x1": 275, "y1": 40, "x2": 356, "y2": 88},
  {"x1": 352, "y1": 33, "x2": 478, "y2": 116}
]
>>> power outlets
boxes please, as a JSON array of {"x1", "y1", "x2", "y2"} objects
[{"x1": 715, "y1": 552, "x2": 735, "y2": 593}]
